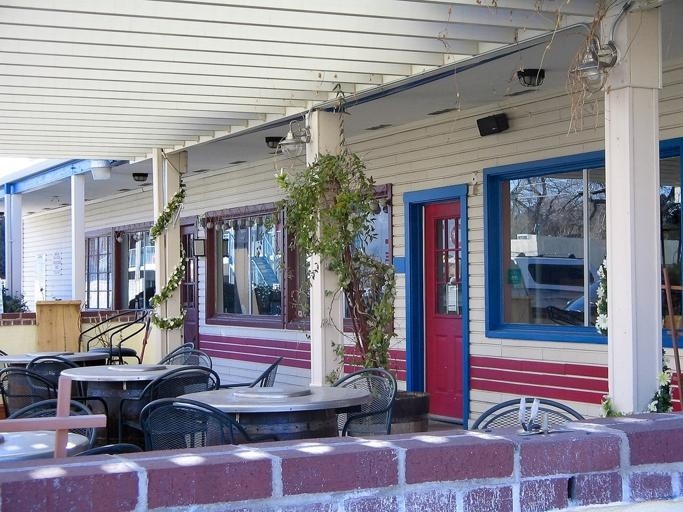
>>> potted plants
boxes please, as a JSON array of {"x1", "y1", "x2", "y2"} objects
[{"x1": 264, "y1": 145, "x2": 430, "y2": 432}]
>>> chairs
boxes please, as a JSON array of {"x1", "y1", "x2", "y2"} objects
[
  {"x1": 0, "y1": 312, "x2": 395, "y2": 466},
  {"x1": 470, "y1": 397, "x2": 588, "y2": 431}
]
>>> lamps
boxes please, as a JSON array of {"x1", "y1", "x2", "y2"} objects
[
  {"x1": 569, "y1": 34, "x2": 617, "y2": 95},
  {"x1": 278, "y1": 120, "x2": 311, "y2": 160}
]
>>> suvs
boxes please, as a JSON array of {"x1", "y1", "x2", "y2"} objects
[{"x1": 513, "y1": 252, "x2": 599, "y2": 327}]
